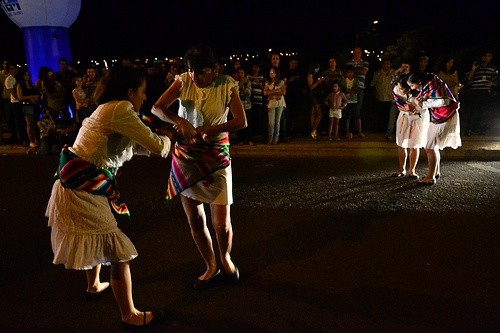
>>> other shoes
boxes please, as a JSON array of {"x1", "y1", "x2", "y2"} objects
[
  {"x1": 325, "y1": 133, "x2": 366, "y2": 142},
  {"x1": 416, "y1": 173, "x2": 440, "y2": 186},
  {"x1": 392, "y1": 170, "x2": 420, "y2": 178},
  {"x1": 84, "y1": 281, "x2": 112, "y2": 297},
  {"x1": 120, "y1": 310, "x2": 160, "y2": 329},
  {"x1": 230, "y1": 136, "x2": 281, "y2": 148}
]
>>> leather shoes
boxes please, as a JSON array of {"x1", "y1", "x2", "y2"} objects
[{"x1": 194, "y1": 266, "x2": 240, "y2": 283}]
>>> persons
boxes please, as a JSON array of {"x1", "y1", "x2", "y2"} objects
[
  {"x1": 407, "y1": 72, "x2": 462, "y2": 185},
  {"x1": 392, "y1": 75, "x2": 431, "y2": 180},
  {"x1": 0, "y1": 46, "x2": 500, "y2": 150},
  {"x1": 151, "y1": 47, "x2": 248, "y2": 286},
  {"x1": 44, "y1": 63, "x2": 166, "y2": 332}
]
